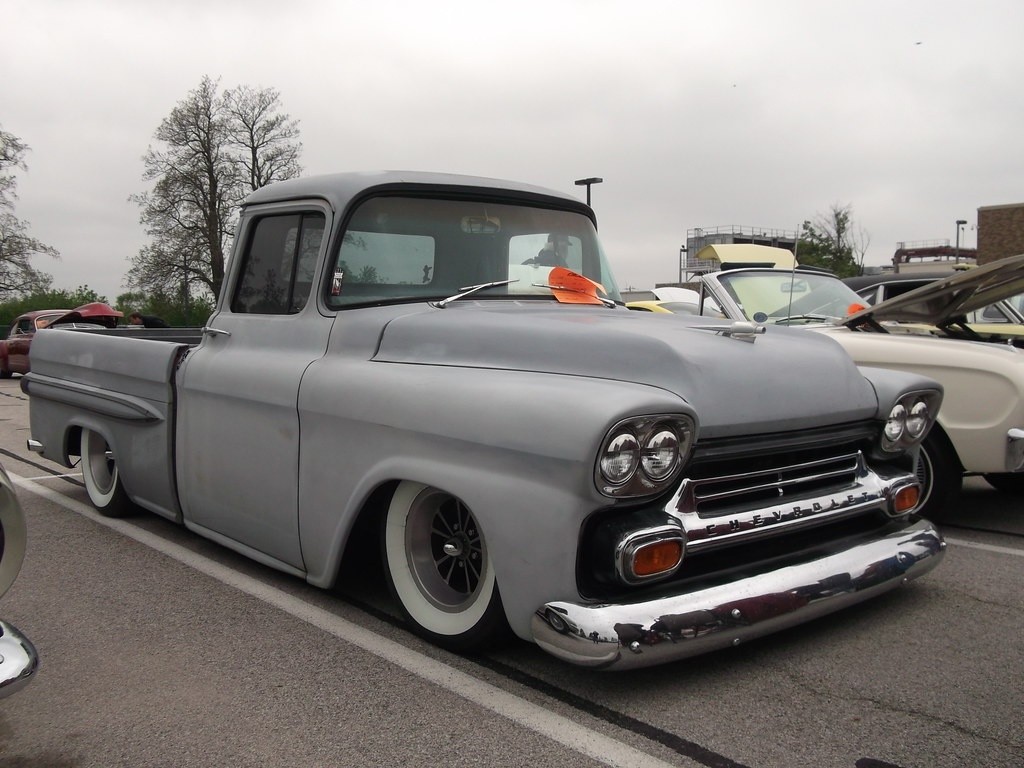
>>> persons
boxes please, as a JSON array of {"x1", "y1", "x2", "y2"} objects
[
  {"x1": 129, "y1": 312, "x2": 170, "y2": 328},
  {"x1": 523, "y1": 233, "x2": 573, "y2": 266}
]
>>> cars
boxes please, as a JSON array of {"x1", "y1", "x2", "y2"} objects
[
  {"x1": 766, "y1": 252, "x2": 1023, "y2": 324},
  {"x1": 0, "y1": 301, "x2": 124, "y2": 380},
  {"x1": 693, "y1": 242, "x2": 1024, "y2": 348},
  {"x1": 624, "y1": 287, "x2": 743, "y2": 318}
]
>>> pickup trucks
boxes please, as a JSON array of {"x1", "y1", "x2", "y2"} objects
[{"x1": 20, "y1": 169, "x2": 947, "y2": 677}]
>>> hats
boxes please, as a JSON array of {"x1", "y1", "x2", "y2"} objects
[{"x1": 548, "y1": 232, "x2": 572, "y2": 246}]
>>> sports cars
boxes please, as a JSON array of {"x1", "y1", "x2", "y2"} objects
[{"x1": 698, "y1": 266, "x2": 1024, "y2": 512}]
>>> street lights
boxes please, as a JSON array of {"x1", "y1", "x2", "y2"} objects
[
  {"x1": 678, "y1": 248, "x2": 689, "y2": 288},
  {"x1": 573, "y1": 176, "x2": 604, "y2": 207},
  {"x1": 954, "y1": 221, "x2": 968, "y2": 272}
]
